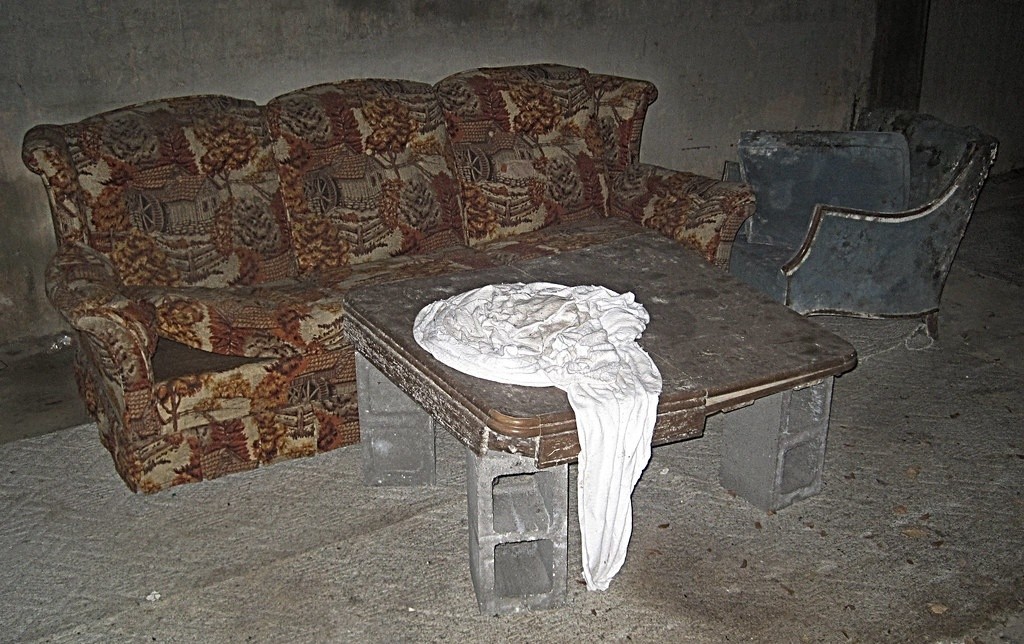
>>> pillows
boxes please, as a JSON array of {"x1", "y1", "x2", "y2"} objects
[{"x1": 732, "y1": 128, "x2": 912, "y2": 246}]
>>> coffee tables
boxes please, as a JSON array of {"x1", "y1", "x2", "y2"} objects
[{"x1": 341, "y1": 230, "x2": 859, "y2": 615}]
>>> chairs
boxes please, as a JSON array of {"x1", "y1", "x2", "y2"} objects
[{"x1": 716, "y1": 107, "x2": 999, "y2": 342}]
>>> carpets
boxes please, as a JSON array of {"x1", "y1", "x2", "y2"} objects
[{"x1": 1, "y1": 265, "x2": 1024, "y2": 642}]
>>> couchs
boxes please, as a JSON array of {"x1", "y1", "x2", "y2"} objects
[{"x1": 23, "y1": 61, "x2": 763, "y2": 496}]
